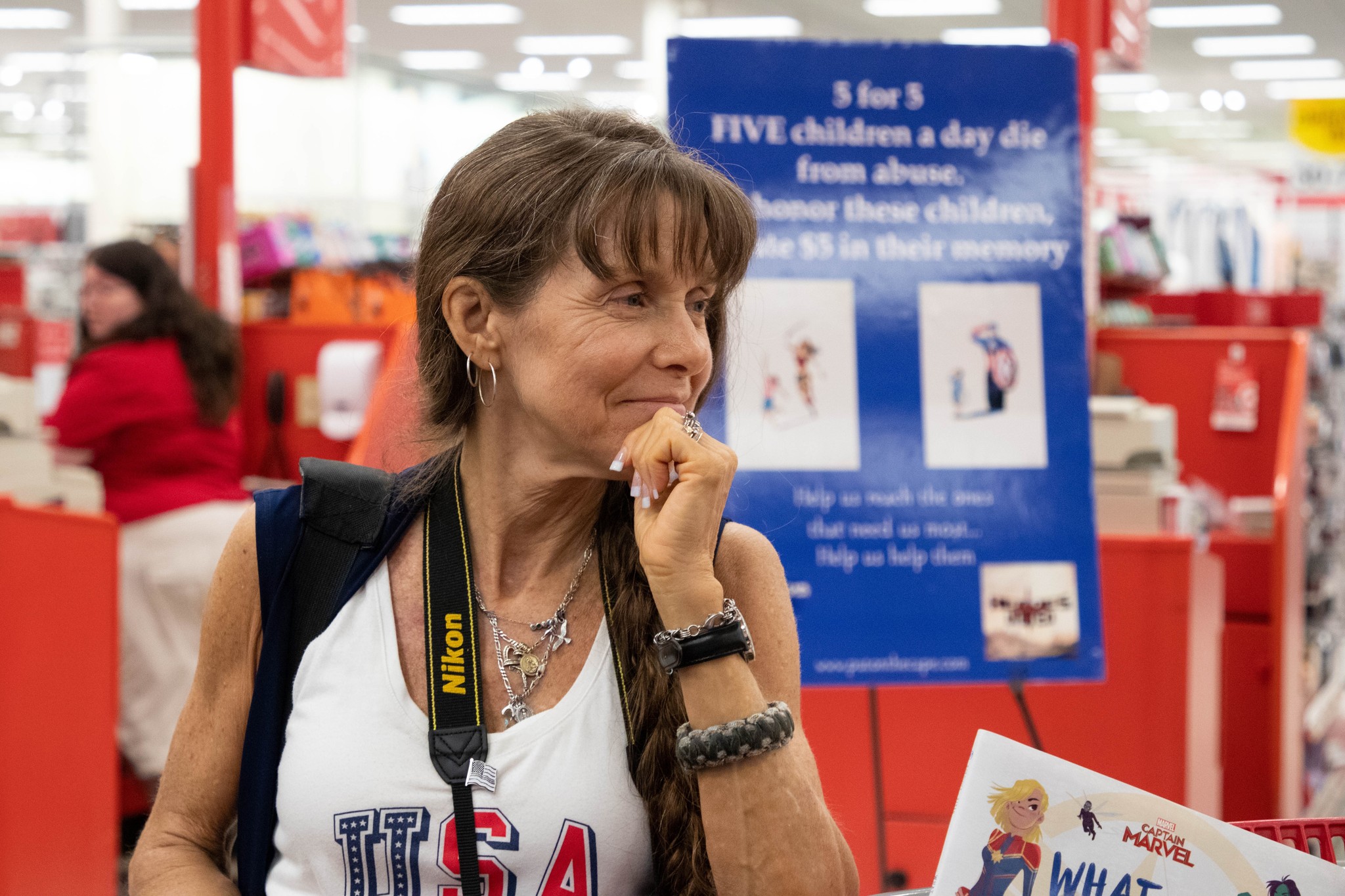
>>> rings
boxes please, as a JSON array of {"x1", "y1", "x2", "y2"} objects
[{"x1": 681, "y1": 410, "x2": 704, "y2": 443}]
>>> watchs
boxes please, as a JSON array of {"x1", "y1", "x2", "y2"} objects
[{"x1": 653, "y1": 600, "x2": 754, "y2": 676}]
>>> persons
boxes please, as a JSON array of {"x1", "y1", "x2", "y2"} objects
[
  {"x1": 41, "y1": 236, "x2": 256, "y2": 878},
  {"x1": 128, "y1": 110, "x2": 862, "y2": 896}
]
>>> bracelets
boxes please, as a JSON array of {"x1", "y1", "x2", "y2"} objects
[
  {"x1": 653, "y1": 597, "x2": 740, "y2": 646},
  {"x1": 676, "y1": 701, "x2": 795, "y2": 770}
]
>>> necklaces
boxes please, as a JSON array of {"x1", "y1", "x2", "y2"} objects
[{"x1": 475, "y1": 528, "x2": 600, "y2": 729}]
